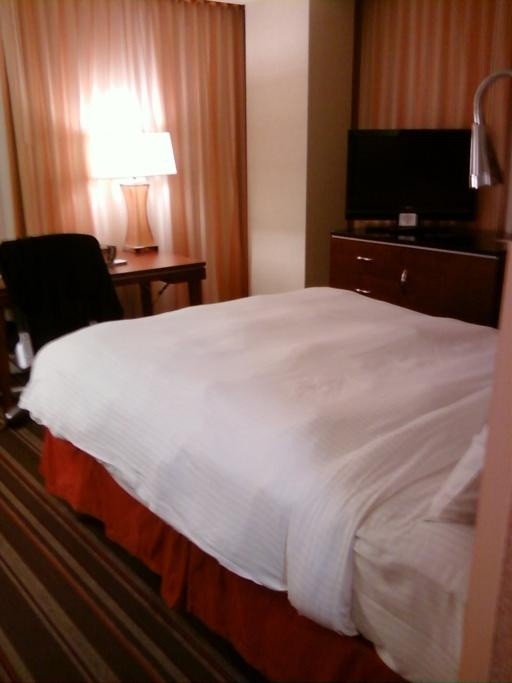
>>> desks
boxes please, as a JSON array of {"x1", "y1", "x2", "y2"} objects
[{"x1": 99, "y1": 250, "x2": 206, "y2": 314}]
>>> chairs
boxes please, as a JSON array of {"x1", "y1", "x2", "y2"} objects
[{"x1": 0, "y1": 234, "x2": 129, "y2": 424}]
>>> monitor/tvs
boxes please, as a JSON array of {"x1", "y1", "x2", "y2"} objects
[{"x1": 345, "y1": 129, "x2": 478, "y2": 242}]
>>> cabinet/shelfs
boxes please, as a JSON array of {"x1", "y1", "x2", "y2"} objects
[{"x1": 326, "y1": 227, "x2": 504, "y2": 330}]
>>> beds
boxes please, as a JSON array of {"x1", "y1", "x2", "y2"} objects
[{"x1": 15, "y1": 249, "x2": 511, "y2": 681}]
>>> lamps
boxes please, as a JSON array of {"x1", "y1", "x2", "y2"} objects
[
  {"x1": 465, "y1": 68, "x2": 512, "y2": 189},
  {"x1": 91, "y1": 131, "x2": 182, "y2": 255}
]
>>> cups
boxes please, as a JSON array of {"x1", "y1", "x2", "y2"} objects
[{"x1": 100, "y1": 244, "x2": 116, "y2": 263}]
